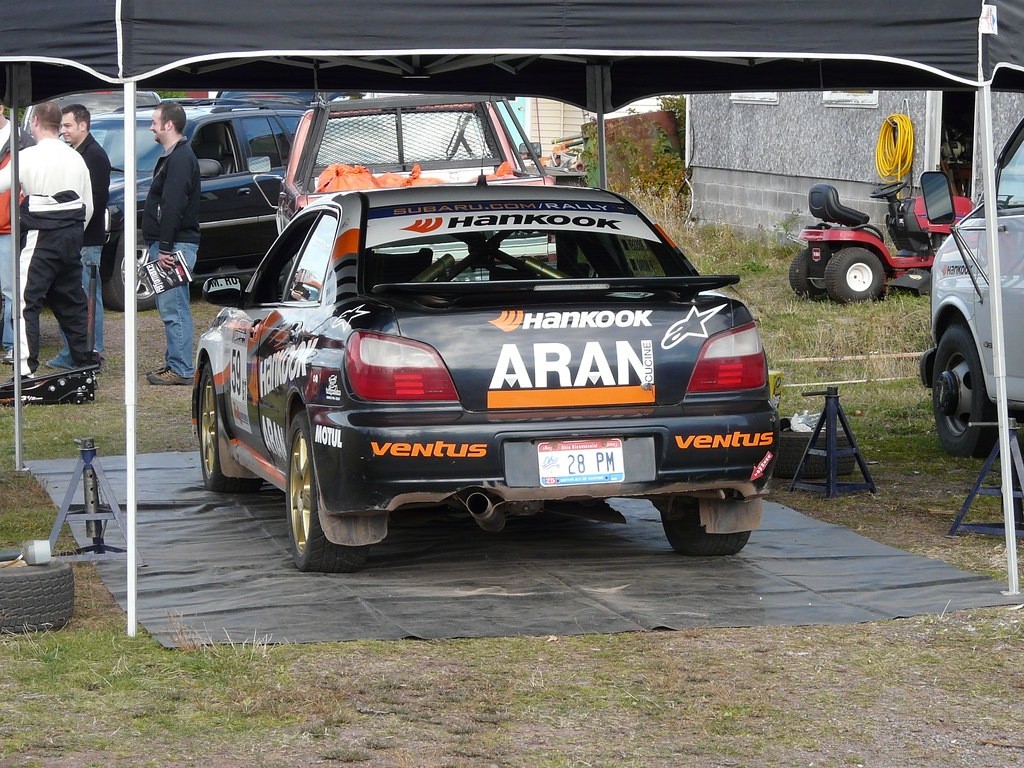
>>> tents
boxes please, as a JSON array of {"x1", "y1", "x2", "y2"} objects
[{"x1": 0, "y1": 0, "x2": 1024, "y2": 635}]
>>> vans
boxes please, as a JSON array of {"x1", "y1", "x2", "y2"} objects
[{"x1": 916, "y1": 103, "x2": 1024, "y2": 458}]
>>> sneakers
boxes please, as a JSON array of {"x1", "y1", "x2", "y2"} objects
[
  {"x1": 146, "y1": 369, "x2": 193, "y2": 385},
  {"x1": 145, "y1": 366, "x2": 171, "y2": 376}
]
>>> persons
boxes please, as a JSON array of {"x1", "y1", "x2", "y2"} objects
[
  {"x1": 0, "y1": 102, "x2": 111, "y2": 381},
  {"x1": 141, "y1": 101, "x2": 200, "y2": 385}
]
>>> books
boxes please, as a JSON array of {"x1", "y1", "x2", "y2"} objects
[{"x1": 144, "y1": 251, "x2": 193, "y2": 295}]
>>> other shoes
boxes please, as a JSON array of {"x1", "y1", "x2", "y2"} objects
[
  {"x1": 7, "y1": 373, "x2": 35, "y2": 384},
  {"x1": 2, "y1": 349, "x2": 14, "y2": 365}
]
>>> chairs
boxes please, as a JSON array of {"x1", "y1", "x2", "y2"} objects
[
  {"x1": 195, "y1": 140, "x2": 235, "y2": 177},
  {"x1": 808, "y1": 182, "x2": 869, "y2": 228}
]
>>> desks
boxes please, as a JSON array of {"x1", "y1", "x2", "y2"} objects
[{"x1": 946, "y1": 162, "x2": 973, "y2": 198}]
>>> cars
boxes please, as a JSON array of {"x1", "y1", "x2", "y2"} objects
[{"x1": 21, "y1": 89, "x2": 557, "y2": 313}]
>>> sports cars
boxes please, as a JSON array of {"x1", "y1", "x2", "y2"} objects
[{"x1": 185, "y1": 179, "x2": 782, "y2": 572}]
